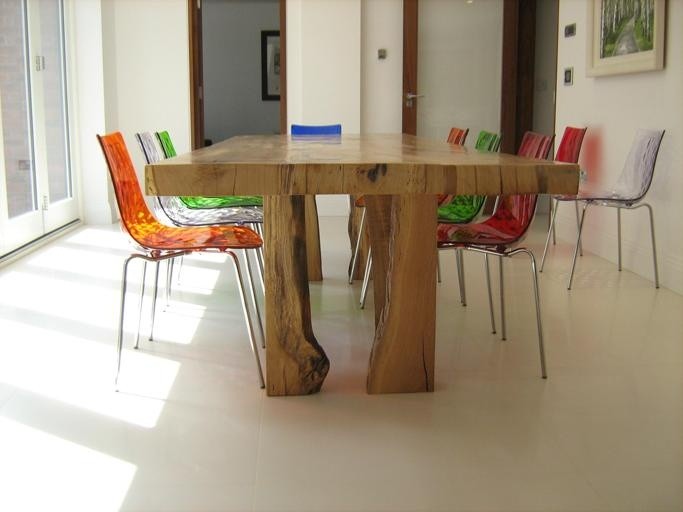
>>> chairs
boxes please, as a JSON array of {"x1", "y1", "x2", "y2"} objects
[
  {"x1": 289, "y1": 125, "x2": 343, "y2": 137},
  {"x1": 349, "y1": 126, "x2": 555, "y2": 380},
  {"x1": 96, "y1": 129, "x2": 267, "y2": 392},
  {"x1": 539, "y1": 125, "x2": 665, "y2": 290}
]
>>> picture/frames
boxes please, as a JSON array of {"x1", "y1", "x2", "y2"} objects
[
  {"x1": 260, "y1": 27, "x2": 284, "y2": 100},
  {"x1": 586, "y1": 0, "x2": 665, "y2": 76}
]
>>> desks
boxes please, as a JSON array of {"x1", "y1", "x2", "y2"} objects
[{"x1": 144, "y1": 133, "x2": 580, "y2": 399}]
